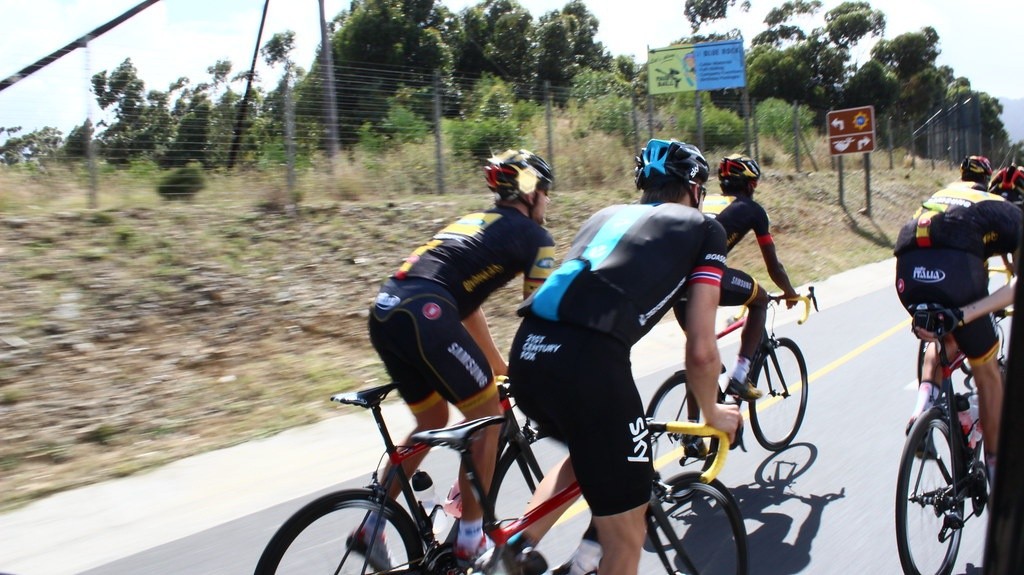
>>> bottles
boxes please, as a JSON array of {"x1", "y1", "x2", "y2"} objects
[
  {"x1": 444, "y1": 481, "x2": 461, "y2": 518},
  {"x1": 959, "y1": 387, "x2": 982, "y2": 450},
  {"x1": 717, "y1": 364, "x2": 736, "y2": 404},
  {"x1": 520, "y1": 547, "x2": 551, "y2": 575},
  {"x1": 568, "y1": 540, "x2": 602, "y2": 575},
  {"x1": 412, "y1": 469, "x2": 447, "y2": 534}
]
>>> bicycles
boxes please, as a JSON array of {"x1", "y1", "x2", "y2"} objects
[
  {"x1": 253, "y1": 376, "x2": 748, "y2": 575},
  {"x1": 645, "y1": 286, "x2": 819, "y2": 504},
  {"x1": 896, "y1": 267, "x2": 1016, "y2": 575}
]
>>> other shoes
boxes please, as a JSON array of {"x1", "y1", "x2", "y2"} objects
[
  {"x1": 466, "y1": 546, "x2": 523, "y2": 575},
  {"x1": 343, "y1": 528, "x2": 392, "y2": 572},
  {"x1": 451, "y1": 535, "x2": 497, "y2": 570},
  {"x1": 727, "y1": 377, "x2": 761, "y2": 401},
  {"x1": 681, "y1": 432, "x2": 709, "y2": 457},
  {"x1": 903, "y1": 418, "x2": 938, "y2": 463}
]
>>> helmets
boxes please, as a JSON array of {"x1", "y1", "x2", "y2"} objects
[
  {"x1": 959, "y1": 154, "x2": 994, "y2": 179},
  {"x1": 718, "y1": 153, "x2": 761, "y2": 186},
  {"x1": 633, "y1": 137, "x2": 710, "y2": 192},
  {"x1": 484, "y1": 147, "x2": 553, "y2": 202},
  {"x1": 988, "y1": 163, "x2": 1024, "y2": 203}
]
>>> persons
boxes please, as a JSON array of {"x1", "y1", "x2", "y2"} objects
[
  {"x1": 939, "y1": 156, "x2": 992, "y2": 191},
  {"x1": 474, "y1": 137, "x2": 743, "y2": 575},
  {"x1": 894, "y1": 166, "x2": 1024, "y2": 511},
  {"x1": 346, "y1": 150, "x2": 556, "y2": 575},
  {"x1": 674, "y1": 153, "x2": 799, "y2": 460}
]
